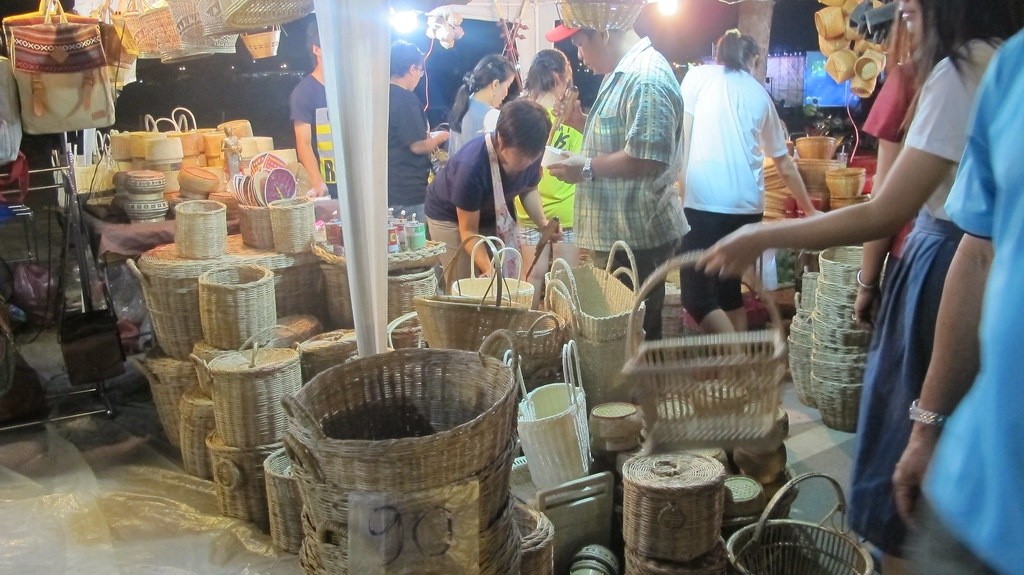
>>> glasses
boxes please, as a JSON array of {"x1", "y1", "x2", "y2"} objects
[{"x1": 415, "y1": 65, "x2": 424, "y2": 77}]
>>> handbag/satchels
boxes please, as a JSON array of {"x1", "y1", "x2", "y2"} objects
[
  {"x1": 4, "y1": 0, "x2": 117, "y2": 136},
  {"x1": 56, "y1": 317, "x2": 126, "y2": 388}
]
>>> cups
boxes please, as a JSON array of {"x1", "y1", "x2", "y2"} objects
[{"x1": 836, "y1": 153, "x2": 848, "y2": 170}]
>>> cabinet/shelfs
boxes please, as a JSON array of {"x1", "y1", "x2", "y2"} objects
[{"x1": 0, "y1": 149, "x2": 115, "y2": 431}]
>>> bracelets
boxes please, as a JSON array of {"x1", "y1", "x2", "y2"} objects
[{"x1": 857, "y1": 269, "x2": 878, "y2": 289}]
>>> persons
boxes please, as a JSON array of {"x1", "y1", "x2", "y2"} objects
[
  {"x1": 422, "y1": 101, "x2": 563, "y2": 300},
  {"x1": 512, "y1": 49, "x2": 583, "y2": 310},
  {"x1": 447, "y1": 52, "x2": 516, "y2": 160},
  {"x1": 288, "y1": 20, "x2": 339, "y2": 200},
  {"x1": 694, "y1": 0, "x2": 1024, "y2": 575},
  {"x1": 681, "y1": 27, "x2": 825, "y2": 383},
  {"x1": 547, "y1": 23, "x2": 691, "y2": 405},
  {"x1": 388, "y1": 40, "x2": 450, "y2": 281}
]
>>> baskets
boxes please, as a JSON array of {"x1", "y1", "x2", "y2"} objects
[
  {"x1": 201, "y1": 338, "x2": 302, "y2": 445},
  {"x1": 622, "y1": 249, "x2": 786, "y2": 431},
  {"x1": 501, "y1": 339, "x2": 594, "y2": 487},
  {"x1": 1, "y1": 1, "x2": 885, "y2": 575},
  {"x1": 728, "y1": 472, "x2": 874, "y2": 575},
  {"x1": 269, "y1": 196, "x2": 318, "y2": 252},
  {"x1": 279, "y1": 329, "x2": 519, "y2": 494},
  {"x1": 198, "y1": 264, "x2": 276, "y2": 346},
  {"x1": 174, "y1": 200, "x2": 228, "y2": 261},
  {"x1": 623, "y1": 452, "x2": 723, "y2": 559},
  {"x1": 559, "y1": 0, "x2": 645, "y2": 34}
]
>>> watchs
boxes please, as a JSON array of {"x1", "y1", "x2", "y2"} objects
[
  {"x1": 908, "y1": 399, "x2": 947, "y2": 427},
  {"x1": 582, "y1": 157, "x2": 594, "y2": 182}
]
